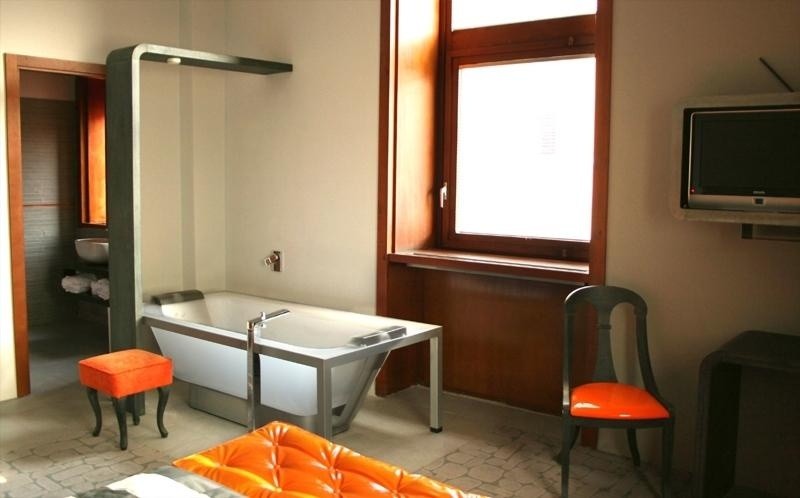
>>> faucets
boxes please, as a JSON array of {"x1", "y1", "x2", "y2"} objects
[{"x1": 246, "y1": 308, "x2": 290, "y2": 337}]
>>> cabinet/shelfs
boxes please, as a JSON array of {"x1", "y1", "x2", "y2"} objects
[{"x1": 45, "y1": 263, "x2": 110, "y2": 307}]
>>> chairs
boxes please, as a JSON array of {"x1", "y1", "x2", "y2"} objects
[{"x1": 555, "y1": 283, "x2": 679, "y2": 497}]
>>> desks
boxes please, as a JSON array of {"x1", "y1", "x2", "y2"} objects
[{"x1": 691, "y1": 328, "x2": 799, "y2": 497}]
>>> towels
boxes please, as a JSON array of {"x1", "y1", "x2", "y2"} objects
[{"x1": 63, "y1": 272, "x2": 110, "y2": 302}]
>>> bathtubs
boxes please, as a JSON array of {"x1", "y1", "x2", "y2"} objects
[{"x1": 142, "y1": 290, "x2": 393, "y2": 417}]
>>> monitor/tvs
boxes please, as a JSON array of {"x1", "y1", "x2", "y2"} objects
[{"x1": 671, "y1": 91, "x2": 800, "y2": 228}]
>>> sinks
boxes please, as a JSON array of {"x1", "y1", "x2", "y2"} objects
[{"x1": 74, "y1": 238, "x2": 110, "y2": 265}]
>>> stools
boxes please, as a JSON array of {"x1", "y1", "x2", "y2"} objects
[{"x1": 76, "y1": 346, "x2": 175, "y2": 452}]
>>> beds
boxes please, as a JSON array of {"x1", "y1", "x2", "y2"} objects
[{"x1": 62, "y1": 420, "x2": 496, "y2": 498}]
